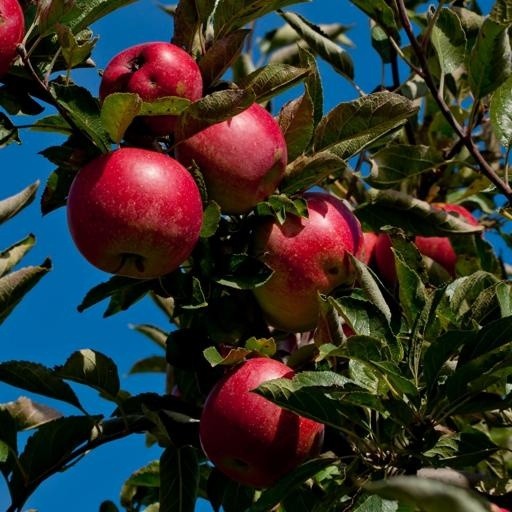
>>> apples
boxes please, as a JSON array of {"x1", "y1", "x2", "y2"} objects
[
  {"x1": 414, "y1": 202, "x2": 482, "y2": 278},
  {"x1": 247, "y1": 192, "x2": 365, "y2": 333},
  {"x1": 0, "y1": 0, "x2": 26, "y2": 76},
  {"x1": 66, "y1": 147, "x2": 203, "y2": 279},
  {"x1": 359, "y1": 229, "x2": 398, "y2": 287},
  {"x1": 287, "y1": 322, "x2": 353, "y2": 368},
  {"x1": 174, "y1": 101, "x2": 288, "y2": 215},
  {"x1": 98, "y1": 41, "x2": 203, "y2": 102},
  {"x1": 199, "y1": 353, "x2": 325, "y2": 488}
]
